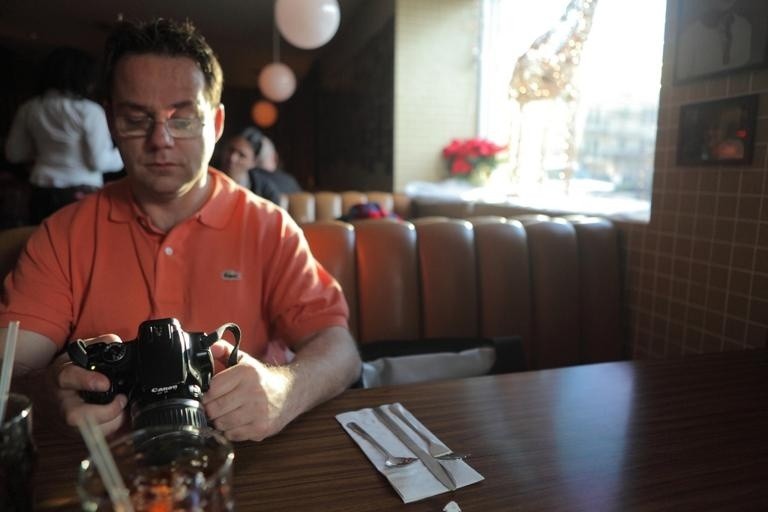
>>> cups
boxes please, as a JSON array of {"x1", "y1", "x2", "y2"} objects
[
  {"x1": 0, "y1": 392, "x2": 38, "y2": 510},
  {"x1": 77, "y1": 426, "x2": 234, "y2": 512}
]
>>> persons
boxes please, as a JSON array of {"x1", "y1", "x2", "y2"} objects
[
  {"x1": 1, "y1": 17, "x2": 363, "y2": 443},
  {"x1": 220, "y1": 126, "x2": 289, "y2": 211},
  {"x1": 4, "y1": 48, "x2": 125, "y2": 225},
  {"x1": 254, "y1": 136, "x2": 303, "y2": 193}
]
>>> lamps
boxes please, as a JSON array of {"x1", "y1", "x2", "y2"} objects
[
  {"x1": 258, "y1": 22, "x2": 296, "y2": 104},
  {"x1": 271, "y1": 0, "x2": 340, "y2": 51}
]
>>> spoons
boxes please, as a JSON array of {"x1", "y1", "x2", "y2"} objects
[{"x1": 346, "y1": 422, "x2": 419, "y2": 468}]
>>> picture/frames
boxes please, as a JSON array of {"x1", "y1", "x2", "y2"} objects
[
  {"x1": 676, "y1": 93, "x2": 757, "y2": 166},
  {"x1": 672, "y1": 1, "x2": 768, "y2": 86}
]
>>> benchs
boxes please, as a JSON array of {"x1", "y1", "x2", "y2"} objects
[
  {"x1": 278, "y1": 191, "x2": 412, "y2": 219},
  {"x1": 1, "y1": 216, "x2": 622, "y2": 388}
]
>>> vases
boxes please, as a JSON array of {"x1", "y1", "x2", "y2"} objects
[{"x1": 465, "y1": 166, "x2": 489, "y2": 186}]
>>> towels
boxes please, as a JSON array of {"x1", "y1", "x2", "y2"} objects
[{"x1": 335, "y1": 406, "x2": 486, "y2": 503}]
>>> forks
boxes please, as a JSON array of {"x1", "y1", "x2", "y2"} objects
[{"x1": 388, "y1": 405, "x2": 472, "y2": 460}]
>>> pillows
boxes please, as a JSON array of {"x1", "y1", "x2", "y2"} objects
[{"x1": 358, "y1": 336, "x2": 496, "y2": 389}]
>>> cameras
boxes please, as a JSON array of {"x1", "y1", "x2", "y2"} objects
[{"x1": 77, "y1": 318, "x2": 243, "y2": 468}]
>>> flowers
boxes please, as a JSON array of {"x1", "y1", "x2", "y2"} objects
[{"x1": 442, "y1": 137, "x2": 507, "y2": 178}]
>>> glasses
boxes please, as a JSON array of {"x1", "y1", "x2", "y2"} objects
[{"x1": 115, "y1": 117, "x2": 205, "y2": 138}]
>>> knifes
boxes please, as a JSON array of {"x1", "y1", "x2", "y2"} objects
[{"x1": 372, "y1": 406, "x2": 456, "y2": 490}]
